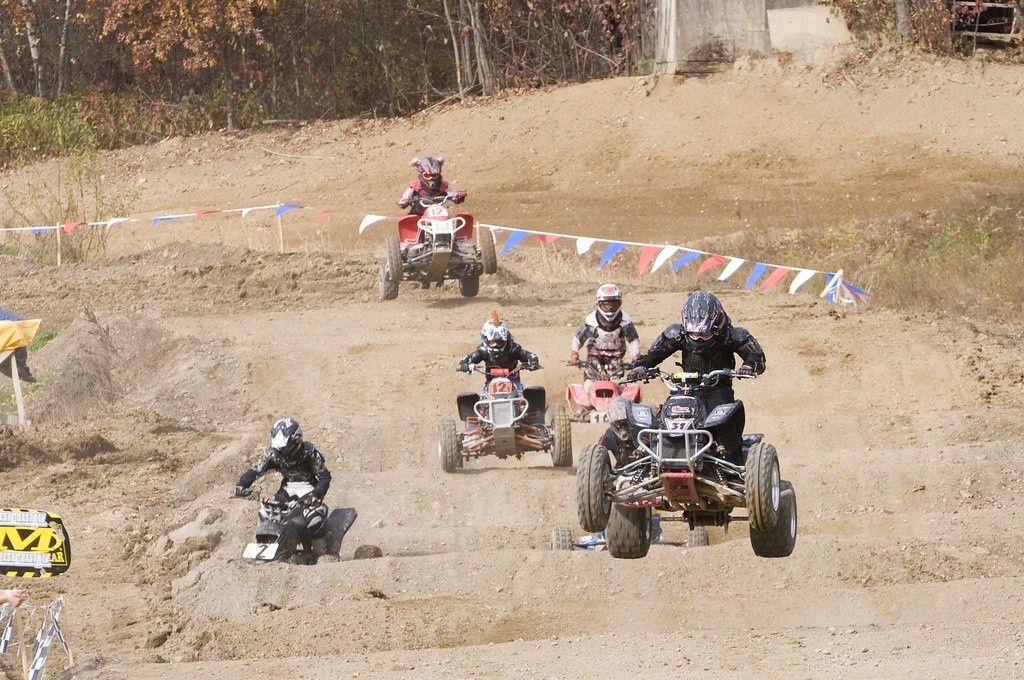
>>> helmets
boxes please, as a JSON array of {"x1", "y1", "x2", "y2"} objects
[
  {"x1": 680, "y1": 291, "x2": 730, "y2": 355},
  {"x1": 410, "y1": 156, "x2": 444, "y2": 196},
  {"x1": 269, "y1": 418, "x2": 304, "y2": 461},
  {"x1": 481, "y1": 318, "x2": 509, "y2": 360},
  {"x1": 595, "y1": 283, "x2": 623, "y2": 332}
]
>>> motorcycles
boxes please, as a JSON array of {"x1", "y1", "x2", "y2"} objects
[
  {"x1": 549, "y1": 516, "x2": 711, "y2": 553},
  {"x1": 575, "y1": 365, "x2": 799, "y2": 560},
  {"x1": 228, "y1": 487, "x2": 383, "y2": 567},
  {"x1": 565, "y1": 358, "x2": 644, "y2": 423},
  {"x1": 377, "y1": 192, "x2": 499, "y2": 300},
  {"x1": 435, "y1": 360, "x2": 575, "y2": 472}
]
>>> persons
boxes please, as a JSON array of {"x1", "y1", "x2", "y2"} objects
[
  {"x1": 459, "y1": 319, "x2": 539, "y2": 423},
  {"x1": 570, "y1": 284, "x2": 642, "y2": 421},
  {"x1": 399, "y1": 157, "x2": 468, "y2": 260},
  {"x1": 628, "y1": 290, "x2": 766, "y2": 485},
  {"x1": 235, "y1": 416, "x2": 332, "y2": 559}
]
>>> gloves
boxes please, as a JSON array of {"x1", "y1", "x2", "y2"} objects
[
  {"x1": 460, "y1": 361, "x2": 469, "y2": 372},
  {"x1": 627, "y1": 365, "x2": 647, "y2": 383},
  {"x1": 397, "y1": 200, "x2": 410, "y2": 208},
  {"x1": 528, "y1": 363, "x2": 539, "y2": 371},
  {"x1": 570, "y1": 351, "x2": 580, "y2": 365},
  {"x1": 234, "y1": 486, "x2": 253, "y2": 498},
  {"x1": 736, "y1": 365, "x2": 753, "y2": 380},
  {"x1": 630, "y1": 353, "x2": 640, "y2": 369},
  {"x1": 303, "y1": 496, "x2": 322, "y2": 508},
  {"x1": 454, "y1": 191, "x2": 467, "y2": 204}
]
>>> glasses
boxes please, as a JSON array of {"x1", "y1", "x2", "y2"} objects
[
  {"x1": 600, "y1": 301, "x2": 620, "y2": 311},
  {"x1": 488, "y1": 341, "x2": 504, "y2": 348}
]
